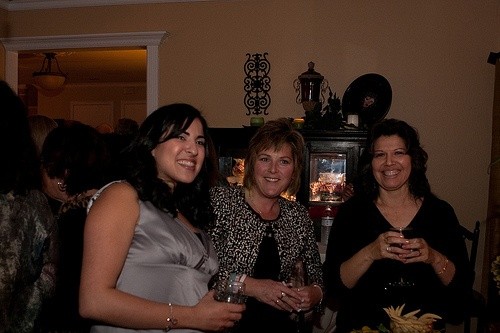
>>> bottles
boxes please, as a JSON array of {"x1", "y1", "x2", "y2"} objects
[{"x1": 321, "y1": 205, "x2": 334, "y2": 245}]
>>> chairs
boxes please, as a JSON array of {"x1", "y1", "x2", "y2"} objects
[{"x1": 460, "y1": 220, "x2": 484, "y2": 333}]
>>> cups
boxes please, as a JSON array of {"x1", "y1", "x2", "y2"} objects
[
  {"x1": 281, "y1": 276, "x2": 304, "y2": 308},
  {"x1": 390, "y1": 225, "x2": 412, "y2": 261},
  {"x1": 348, "y1": 115, "x2": 358, "y2": 127},
  {"x1": 214, "y1": 279, "x2": 247, "y2": 304}
]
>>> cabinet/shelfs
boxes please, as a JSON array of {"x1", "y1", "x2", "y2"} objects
[{"x1": 208, "y1": 127, "x2": 368, "y2": 208}]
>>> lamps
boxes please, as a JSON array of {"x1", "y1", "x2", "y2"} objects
[{"x1": 32, "y1": 52, "x2": 66, "y2": 91}]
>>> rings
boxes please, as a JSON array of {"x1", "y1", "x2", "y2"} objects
[
  {"x1": 280, "y1": 292, "x2": 287, "y2": 300},
  {"x1": 386, "y1": 246, "x2": 389, "y2": 252},
  {"x1": 275, "y1": 299, "x2": 280, "y2": 304}
]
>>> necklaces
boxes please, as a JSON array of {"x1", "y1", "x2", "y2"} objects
[{"x1": 247, "y1": 190, "x2": 282, "y2": 221}]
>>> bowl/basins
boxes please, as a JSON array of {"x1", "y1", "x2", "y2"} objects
[{"x1": 390, "y1": 319, "x2": 433, "y2": 333}]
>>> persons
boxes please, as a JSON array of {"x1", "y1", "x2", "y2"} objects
[
  {"x1": 0, "y1": 78, "x2": 145, "y2": 333},
  {"x1": 203, "y1": 122, "x2": 326, "y2": 333},
  {"x1": 78, "y1": 98, "x2": 247, "y2": 332},
  {"x1": 324, "y1": 119, "x2": 475, "y2": 333}
]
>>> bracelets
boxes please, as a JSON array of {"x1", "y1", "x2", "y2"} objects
[
  {"x1": 435, "y1": 256, "x2": 449, "y2": 276},
  {"x1": 237, "y1": 273, "x2": 247, "y2": 296},
  {"x1": 161, "y1": 302, "x2": 177, "y2": 332}
]
